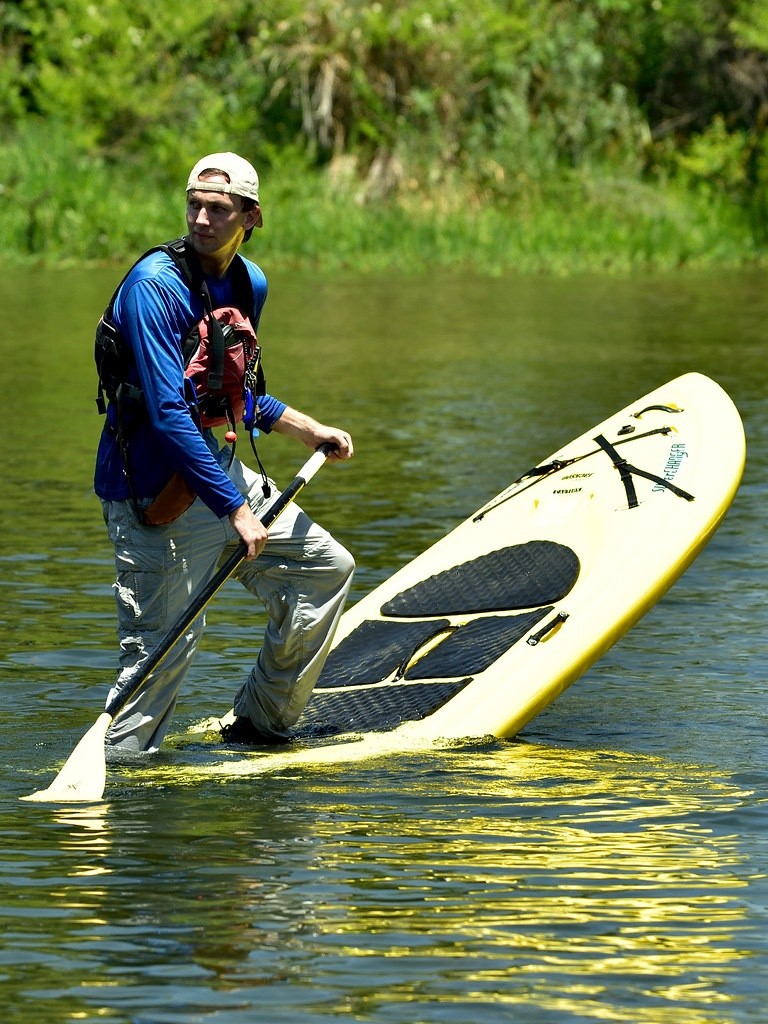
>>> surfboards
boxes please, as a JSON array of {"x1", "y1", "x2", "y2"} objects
[{"x1": 222, "y1": 371, "x2": 746, "y2": 746}]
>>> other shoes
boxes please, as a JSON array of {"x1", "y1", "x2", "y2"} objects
[{"x1": 219, "y1": 715, "x2": 297, "y2": 746}]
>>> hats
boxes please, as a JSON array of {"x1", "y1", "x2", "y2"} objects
[{"x1": 185, "y1": 150, "x2": 264, "y2": 230}]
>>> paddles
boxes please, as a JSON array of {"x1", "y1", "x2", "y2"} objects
[{"x1": 50, "y1": 440, "x2": 341, "y2": 792}]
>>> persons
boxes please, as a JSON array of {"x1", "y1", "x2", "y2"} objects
[{"x1": 93, "y1": 151, "x2": 356, "y2": 753}]
